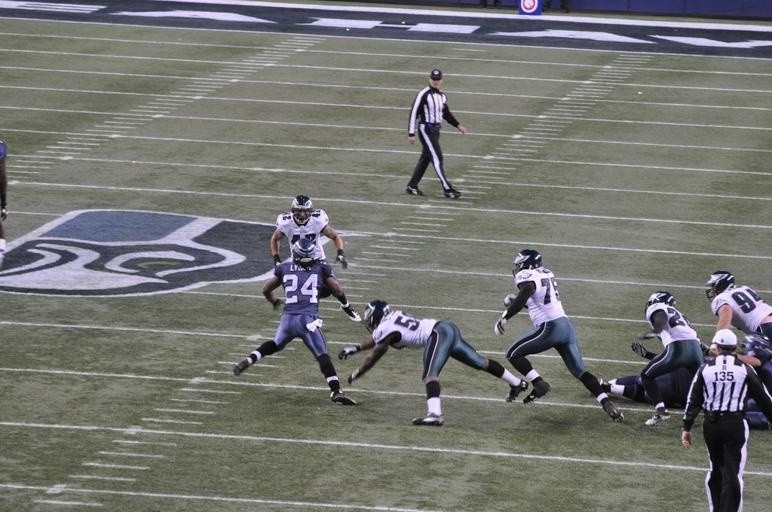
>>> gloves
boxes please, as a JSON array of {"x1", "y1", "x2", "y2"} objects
[
  {"x1": 494, "y1": 317, "x2": 507, "y2": 337},
  {"x1": 348, "y1": 367, "x2": 362, "y2": 384},
  {"x1": 334, "y1": 255, "x2": 347, "y2": 269},
  {"x1": 339, "y1": 345, "x2": 360, "y2": 360}
]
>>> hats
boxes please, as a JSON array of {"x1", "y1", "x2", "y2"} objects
[{"x1": 430, "y1": 69, "x2": 442, "y2": 79}]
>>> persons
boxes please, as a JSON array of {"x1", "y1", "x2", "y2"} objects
[
  {"x1": 598, "y1": 271, "x2": 772, "y2": 430},
  {"x1": 405, "y1": 69, "x2": 466, "y2": 199},
  {"x1": 262, "y1": 196, "x2": 357, "y2": 323},
  {"x1": 494, "y1": 249, "x2": 624, "y2": 424},
  {"x1": 0, "y1": 138, "x2": 7, "y2": 253},
  {"x1": 337, "y1": 300, "x2": 529, "y2": 427},
  {"x1": 233, "y1": 239, "x2": 357, "y2": 406},
  {"x1": 681, "y1": 329, "x2": 772, "y2": 512}
]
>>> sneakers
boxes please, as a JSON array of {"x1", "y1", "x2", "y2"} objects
[
  {"x1": 413, "y1": 413, "x2": 444, "y2": 426},
  {"x1": 330, "y1": 389, "x2": 356, "y2": 405},
  {"x1": 233, "y1": 359, "x2": 250, "y2": 376},
  {"x1": 405, "y1": 185, "x2": 422, "y2": 195},
  {"x1": 341, "y1": 302, "x2": 361, "y2": 322},
  {"x1": 444, "y1": 189, "x2": 460, "y2": 198},
  {"x1": 507, "y1": 378, "x2": 671, "y2": 426}
]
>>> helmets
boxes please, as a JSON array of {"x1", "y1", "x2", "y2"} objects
[
  {"x1": 645, "y1": 270, "x2": 770, "y2": 355},
  {"x1": 292, "y1": 239, "x2": 315, "y2": 267},
  {"x1": 364, "y1": 300, "x2": 391, "y2": 333},
  {"x1": 291, "y1": 195, "x2": 313, "y2": 226},
  {"x1": 512, "y1": 249, "x2": 541, "y2": 277}
]
